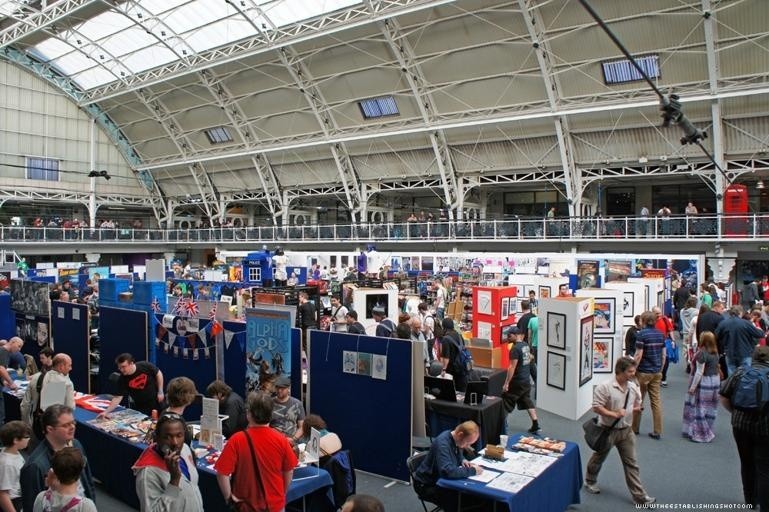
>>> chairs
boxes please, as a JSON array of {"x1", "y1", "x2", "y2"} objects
[
  {"x1": 407, "y1": 451, "x2": 440, "y2": 512},
  {"x1": 324, "y1": 449, "x2": 357, "y2": 512}
]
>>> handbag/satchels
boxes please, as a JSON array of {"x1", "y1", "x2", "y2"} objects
[
  {"x1": 582, "y1": 415, "x2": 613, "y2": 451},
  {"x1": 665, "y1": 338, "x2": 679, "y2": 363},
  {"x1": 32, "y1": 410, "x2": 46, "y2": 438}
]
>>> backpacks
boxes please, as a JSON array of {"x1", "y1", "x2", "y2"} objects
[
  {"x1": 731, "y1": 361, "x2": 769, "y2": 410},
  {"x1": 423, "y1": 315, "x2": 443, "y2": 338},
  {"x1": 377, "y1": 321, "x2": 397, "y2": 338},
  {"x1": 441, "y1": 334, "x2": 474, "y2": 375}
]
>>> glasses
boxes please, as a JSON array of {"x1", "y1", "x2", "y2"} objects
[{"x1": 159, "y1": 414, "x2": 183, "y2": 421}]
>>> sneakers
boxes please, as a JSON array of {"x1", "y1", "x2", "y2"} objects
[
  {"x1": 528, "y1": 428, "x2": 542, "y2": 433},
  {"x1": 632, "y1": 496, "x2": 655, "y2": 506},
  {"x1": 584, "y1": 481, "x2": 601, "y2": 494}
]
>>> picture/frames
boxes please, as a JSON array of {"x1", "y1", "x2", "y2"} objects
[
  {"x1": 623, "y1": 291, "x2": 635, "y2": 318},
  {"x1": 592, "y1": 336, "x2": 615, "y2": 374},
  {"x1": 546, "y1": 350, "x2": 568, "y2": 391},
  {"x1": 509, "y1": 296, "x2": 519, "y2": 315},
  {"x1": 500, "y1": 296, "x2": 510, "y2": 322},
  {"x1": 643, "y1": 285, "x2": 651, "y2": 313},
  {"x1": 546, "y1": 310, "x2": 568, "y2": 351},
  {"x1": 538, "y1": 285, "x2": 553, "y2": 300},
  {"x1": 623, "y1": 324, "x2": 634, "y2": 351},
  {"x1": 517, "y1": 296, "x2": 530, "y2": 313},
  {"x1": 594, "y1": 296, "x2": 617, "y2": 335},
  {"x1": 499, "y1": 324, "x2": 511, "y2": 344},
  {"x1": 579, "y1": 314, "x2": 594, "y2": 387}
]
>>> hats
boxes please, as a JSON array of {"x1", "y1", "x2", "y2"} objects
[
  {"x1": 372, "y1": 302, "x2": 385, "y2": 313},
  {"x1": 275, "y1": 377, "x2": 291, "y2": 388},
  {"x1": 502, "y1": 326, "x2": 520, "y2": 334}
]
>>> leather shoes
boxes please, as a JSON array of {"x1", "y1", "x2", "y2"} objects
[{"x1": 647, "y1": 432, "x2": 660, "y2": 440}]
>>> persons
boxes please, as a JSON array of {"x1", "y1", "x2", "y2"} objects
[
  {"x1": 714, "y1": 304, "x2": 767, "y2": 377},
  {"x1": 742, "y1": 275, "x2": 769, "y2": 348},
  {"x1": 166, "y1": 263, "x2": 222, "y2": 303},
  {"x1": 527, "y1": 290, "x2": 538, "y2": 314},
  {"x1": 39, "y1": 347, "x2": 55, "y2": 374},
  {"x1": 25, "y1": 216, "x2": 89, "y2": 240},
  {"x1": 289, "y1": 414, "x2": 343, "y2": 468},
  {"x1": 0, "y1": 336, "x2": 23, "y2": 427},
  {"x1": 657, "y1": 206, "x2": 672, "y2": 235},
  {"x1": 0, "y1": 420, "x2": 34, "y2": 512},
  {"x1": 195, "y1": 220, "x2": 246, "y2": 239},
  {"x1": 131, "y1": 412, "x2": 205, "y2": 512},
  {"x1": 625, "y1": 315, "x2": 642, "y2": 357},
  {"x1": 555, "y1": 284, "x2": 572, "y2": 299},
  {"x1": 0, "y1": 340, "x2": 26, "y2": 376},
  {"x1": 682, "y1": 331, "x2": 721, "y2": 443},
  {"x1": 259, "y1": 249, "x2": 273, "y2": 287},
  {"x1": 95, "y1": 352, "x2": 165, "y2": 420},
  {"x1": 704, "y1": 278, "x2": 726, "y2": 331},
  {"x1": 20, "y1": 352, "x2": 76, "y2": 455},
  {"x1": 272, "y1": 246, "x2": 290, "y2": 286},
  {"x1": 516, "y1": 301, "x2": 536, "y2": 384},
  {"x1": 341, "y1": 494, "x2": 385, "y2": 512},
  {"x1": 719, "y1": 345, "x2": 769, "y2": 512},
  {"x1": 547, "y1": 207, "x2": 556, "y2": 236},
  {"x1": 38, "y1": 321, "x2": 48, "y2": 346},
  {"x1": 684, "y1": 204, "x2": 698, "y2": 234},
  {"x1": 159, "y1": 377, "x2": 198, "y2": 464},
  {"x1": 584, "y1": 357, "x2": 656, "y2": 506},
  {"x1": 33, "y1": 446, "x2": 97, "y2": 512},
  {"x1": 527, "y1": 316, "x2": 537, "y2": 364},
  {"x1": 501, "y1": 326, "x2": 542, "y2": 433},
  {"x1": 206, "y1": 380, "x2": 249, "y2": 441},
  {"x1": 49, "y1": 271, "x2": 103, "y2": 374},
  {"x1": 671, "y1": 278, "x2": 704, "y2": 373},
  {"x1": 412, "y1": 420, "x2": 484, "y2": 512},
  {"x1": 20, "y1": 404, "x2": 96, "y2": 512},
  {"x1": 633, "y1": 312, "x2": 667, "y2": 441},
  {"x1": 639, "y1": 205, "x2": 649, "y2": 238},
  {"x1": 652, "y1": 306, "x2": 676, "y2": 388},
  {"x1": 213, "y1": 395, "x2": 299, "y2": 512},
  {"x1": 289, "y1": 263, "x2": 473, "y2": 393},
  {"x1": 269, "y1": 377, "x2": 306, "y2": 459},
  {"x1": 94, "y1": 218, "x2": 121, "y2": 239},
  {"x1": 407, "y1": 209, "x2": 449, "y2": 237}
]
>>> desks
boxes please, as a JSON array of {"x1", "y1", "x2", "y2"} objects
[
  {"x1": 436, "y1": 433, "x2": 583, "y2": 512},
  {"x1": 2, "y1": 371, "x2": 334, "y2": 512},
  {"x1": 425, "y1": 391, "x2": 508, "y2": 450}
]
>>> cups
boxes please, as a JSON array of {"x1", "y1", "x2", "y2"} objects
[{"x1": 499, "y1": 435, "x2": 508, "y2": 448}]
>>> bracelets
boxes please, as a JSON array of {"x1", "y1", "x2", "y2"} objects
[
  {"x1": 157, "y1": 390, "x2": 163, "y2": 394},
  {"x1": 293, "y1": 436, "x2": 300, "y2": 442}
]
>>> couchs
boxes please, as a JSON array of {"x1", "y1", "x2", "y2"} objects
[{"x1": 429, "y1": 361, "x2": 507, "y2": 395}]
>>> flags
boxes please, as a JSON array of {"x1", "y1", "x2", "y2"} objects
[
  {"x1": 183, "y1": 301, "x2": 200, "y2": 317},
  {"x1": 207, "y1": 301, "x2": 217, "y2": 319},
  {"x1": 174, "y1": 297, "x2": 187, "y2": 315},
  {"x1": 150, "y1": 296, "x2": 161, "y2": 314}
]
